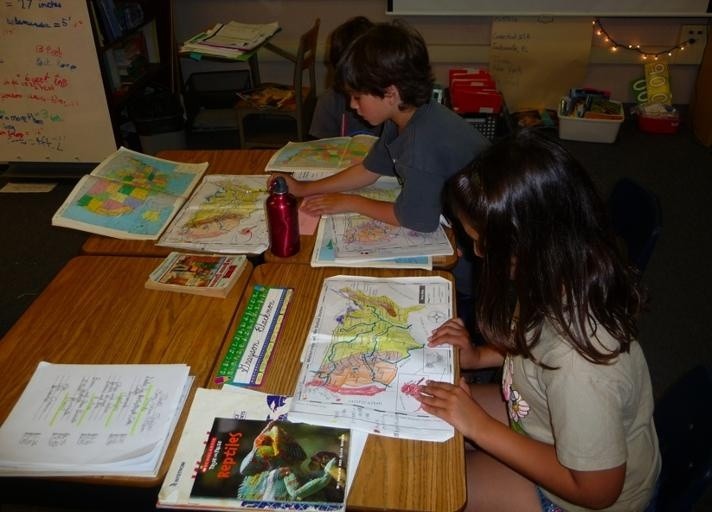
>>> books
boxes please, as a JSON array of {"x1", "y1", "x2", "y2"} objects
[
  {"x1": 144, "y1": 253, "x2": 248, "y2": 299},
  {"x1": 311, "y1": 176, "x2": 454, "y2": 272},
  {"x1": 264, "y1": 134, "x2": 379, "y2": 172},
  {"x1": 51, "y1": 145, "x2": 209, "y2": 242},
  {"x1": 0, "y1": 360, "x2": 196, "y2": 478},
  {"x1": 155, "y1": 387, "x2": 371, "y2": 512}
]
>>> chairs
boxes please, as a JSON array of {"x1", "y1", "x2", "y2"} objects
[{"x1": 238, "y1": 18, "x2": 322, "y2": 148}]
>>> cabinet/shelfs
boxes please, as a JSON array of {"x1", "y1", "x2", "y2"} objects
[{"x1": 78, "y1": 0, "x2": 189, "y2": 153}]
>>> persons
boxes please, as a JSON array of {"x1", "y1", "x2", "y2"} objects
[
  {"x1": 268, "y1": 19, "x2": 495, "y2": 233},
  {"x1": 308, "y1": 17, "x2": 383, "y2": 139},
  {"x1": 419, "y1": 139, "x2": 662, "y2": 511}
]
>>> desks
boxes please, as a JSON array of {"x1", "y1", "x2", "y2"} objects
[
  {"x1": 82, "y1": 147, "x2": 273, "y2": 263},
  {"x1": 174, "y1": 263, "x2": 469, "y2": 511},
  {"x1": 264, "y1": 140, "x2": 461, "y2": 270},
  {"x1": 0, "y1": 256, "x2": 256, "y2": 494}
]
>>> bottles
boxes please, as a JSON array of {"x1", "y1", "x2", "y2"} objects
[{"x1": 266, "y1": 177, "x2": 300, "y2": 258}]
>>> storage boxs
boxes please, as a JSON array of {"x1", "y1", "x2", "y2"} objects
[{"x1": 556, "y1": 98, "x2": 625, "y2": 143}]
